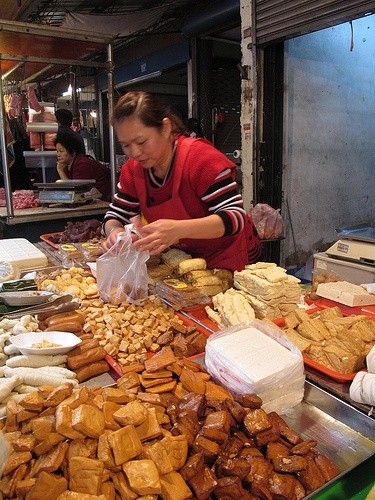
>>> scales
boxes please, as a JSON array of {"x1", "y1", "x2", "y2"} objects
[
  {"x1": 39, "y1": 179, "x2": 96, "y2": 207},
  {"x1": 325, "y1": 226, "x2": 375, "y2": 266}
]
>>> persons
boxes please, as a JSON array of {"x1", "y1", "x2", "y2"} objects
[
  {"x1": 102, "y1": 90, "x2": 265, "y2": 271},
  {"x1": 54, "y1": 108, "x2": 86, "y2": 156},
  {"x1": 53, "y1": 131, "x2": 119, "y2": 202}
]
